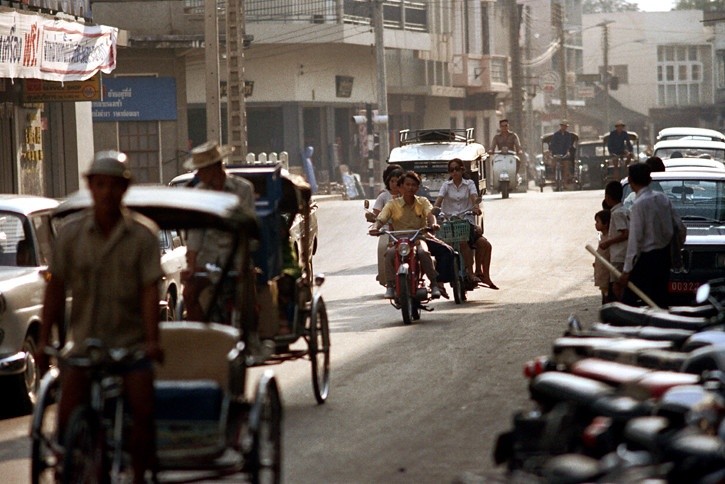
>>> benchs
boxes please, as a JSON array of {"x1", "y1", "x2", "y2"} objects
[{"x1": 152, "y1": 321, "x2": 243, "y2": 423}]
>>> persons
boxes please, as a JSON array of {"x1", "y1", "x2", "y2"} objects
[
  {"x1": 178, "y1": 141, "x2": 280, "y2": 366},
  {"x1": 608, "y1": 119, "x2": 633, "y2": 168},
  {"x1": 489, "y1": 120, "x2": 523, "y2": 182},
  {"x1": 34, "y1": 149, "x2": 164, "y2": 484},
  {"x1": 548, "y1": 120, "x2": 571, "y2": 160},
  {"x1": 369, "y1": 158, "x2": 500, "y2": 300},
  {"x1": 593, "y1": 156, "x2": 687, "y2": 311}
]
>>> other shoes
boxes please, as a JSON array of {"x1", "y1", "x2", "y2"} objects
[
  {"x1": 384, "y1": 286, "x2": 395, "y2": 297},
  {"x1": 431, "y1": 283, "x2": 450, "y2": 299},
  {"x1": 469, "y1": 274, "x2": 481, "y2": 282}
]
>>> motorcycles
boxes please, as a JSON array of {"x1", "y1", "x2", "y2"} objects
[
  {"x1": 491, "y1": 283, "x2": 725, "y2": 484},
  {"x1": 364, "y1": 200, "x2": 441, "y2": 324}
]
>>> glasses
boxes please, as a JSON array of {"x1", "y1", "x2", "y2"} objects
[
  {"x1": 501, "y1": 124, "x2": 508, "y2": 127},
  {"x1": 450, "y1": 166, "x2": 464, "y2": 172}
]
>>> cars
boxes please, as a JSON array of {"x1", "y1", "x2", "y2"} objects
[
  {"x1": 578, "y1": 142, "x2": 610, "y2": 189},
  {"x1": 534, "y1": 154, "x2": 546, "y2": 186},
  {"x1": 619, "y1": 172, "x2": 725, "y2": 303},
  {"x1": 0, "y1": 193, "x2": 179, "y2": 414},
  {"x1": 633, "y1": 145, "x2": 647, "y2": 159},
  {"x1": 652, "y1": 128, "x2": 725, "y2": 225}
]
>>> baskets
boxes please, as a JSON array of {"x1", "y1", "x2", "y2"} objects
[{"x1": 435, "y1": 219, "x2": 470, "y2": 242}]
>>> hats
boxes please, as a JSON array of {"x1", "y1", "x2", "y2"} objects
[
  {"x1": 182, "y1": 141, "x2": 235, "y2": 169},
  {"x1": 83, "y1": 157, "x2": 138, "y2": 180},
  {"x1": 614, "y1": 120, "x2": 625, "y2": 127},
  {"x1": 559, "y1": 119, "x2": 569, "y2": 127}
]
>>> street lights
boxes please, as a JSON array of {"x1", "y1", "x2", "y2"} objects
[{"x1": 603, "y1": 39, "x2": 644, "y2": 135}]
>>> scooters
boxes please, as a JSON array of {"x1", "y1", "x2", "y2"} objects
[
  {"x1": 492, "y1": 147, "x2": 522, "y2": 199},
  {"x1": 438, "y1": 198, "x2": 483, "y2": 304},
  {"x1": 385, "y1": 127, "x2": 489, "y2": 206}
]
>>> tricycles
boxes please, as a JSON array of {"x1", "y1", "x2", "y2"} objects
[
  {"x1": 600, "y1": 132, "x2": 640, "y2": 186},
  {"x1": 167, "y1": 162, "x2": 330, "y2": 404},
  {"x1": 30, "y1": 185, "x2": 283, "y2": 484},
  {"x1": 541, "y1": 133, "x2": 579, "y2": 192}
]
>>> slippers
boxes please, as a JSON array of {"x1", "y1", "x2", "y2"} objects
[{"x1": 478, "y1": 283, "x2": 499, "y2": 290}]
